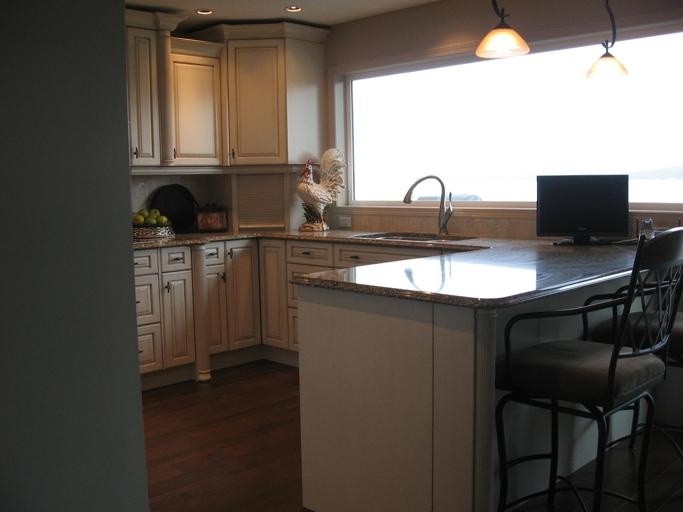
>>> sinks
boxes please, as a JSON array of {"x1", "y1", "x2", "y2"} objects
[{"x1": 352, "y1": 230, "x2": 478, "y2": 242}]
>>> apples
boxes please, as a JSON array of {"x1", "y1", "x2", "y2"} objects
[
  {"x1": 146, "y1": 218, "x2": 157, "y2": 226},
  {"x1": 157, "y1": 215, "x2": 168, "y2": 226},
  {"x1": 149, "y1": 209, "x2": 160, "y2": 218},
  {"x1": 137, "y1": 208, "x2": 150, "y2": 218},
  {"x1": 133, "y1": 215, "x2": 145, "y2": 226}
]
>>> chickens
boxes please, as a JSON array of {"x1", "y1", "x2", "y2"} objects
[{"x1": 296, "y1": 148, "x2": 347, "y2": 231}]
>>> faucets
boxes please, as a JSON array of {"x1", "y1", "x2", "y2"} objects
[{"x1": 403, "y1": 175, "x2": 454, "y2": 234}]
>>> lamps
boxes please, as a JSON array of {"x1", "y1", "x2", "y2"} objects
[
  {"x1": 584, "y1": 0, "x2": 628, "y2": 77},
  {"x1": 474, "y1": 0, "x2": 530, "y2": 60}
]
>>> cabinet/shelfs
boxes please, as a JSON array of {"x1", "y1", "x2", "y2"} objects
[
  {"x1": 160, "y1": 244, "x2": 201, "y2": 370},
  {"x1": 228, "y1": 240, "x2": 262, "y2": 349},
  {"x1": 220, "y1": 33, "x2": 292, "y2": 169},
  {"x1": 127, "y1": 20, "x2": 169, "y2": 172},
  {"x1": 195, "y1": 239, "x2": 225, "y2": 360},
  {"x1": 257, "y1": 239, "x2": 286, "y2": 356},
  {"x1": 167, "y1": 45, "x2": 220, "y2": 167},
  {"x1": 283, "y1": 238, "x2": 454, "y2": 360},
  {"x1": 131, "y1": 247, "x2": 165, "y2": 380}
]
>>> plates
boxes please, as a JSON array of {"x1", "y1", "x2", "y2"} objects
[{"x1": 133, "y1": 226, "x2": 176, "y2": 243}]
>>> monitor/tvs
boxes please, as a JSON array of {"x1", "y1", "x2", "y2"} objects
[{"x1": 537, "y1": 174, "x2": 629, "y2": 248}]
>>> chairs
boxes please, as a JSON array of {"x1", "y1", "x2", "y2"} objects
[
  {"x1": 580, "y1": 275, "x2": 683, "y2": 371},
  {"x1": 492, "y1": 226, "x2": 683, "y2": 512}
]
